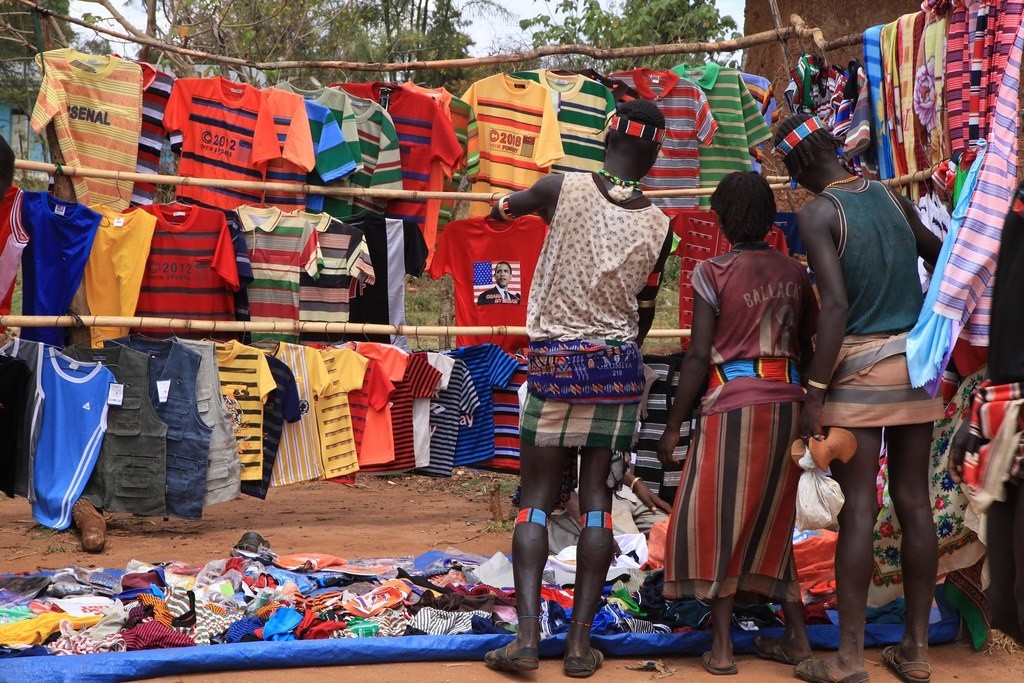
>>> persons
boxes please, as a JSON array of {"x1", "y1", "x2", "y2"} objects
[
  {"x1": 766, "y1": 110, "x2": 946, "y2": 683},
  {"x1": 477, "y1": 261, "x2": 520, "y2": 306},
  {"x1": 946, "y1": 176, "x2": 1024, "y2": 650},
  {"x1": 516, "y1": 378, "x2": 672, "y2": 566},
  {"x1": 484, "y1": 97, "x2": 674, "y2": 677},
  {"x1": 656, "y1": 169, "x2": 820, "y2": 677}
]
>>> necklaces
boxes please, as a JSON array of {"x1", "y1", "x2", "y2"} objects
[
  {"x1": 822, "y1": 175, "x2": 861, "y2": 191},
  {"x1": 599, "y1": 168, "x2": 645, "y2": 191}
]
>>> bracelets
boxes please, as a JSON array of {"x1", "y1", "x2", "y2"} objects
[
  {"x1": 626, "y1": 476, "x2": 643, "y2": 495},
  {"x1": 495, "y1": 193, "x2": 518, "y2": 222},
  {"x1": 807, "y1": 377, "x2": 828, "y2": 391},
  {"x1": 638, "y1": 297, "x2": 657, "y2": 310}
]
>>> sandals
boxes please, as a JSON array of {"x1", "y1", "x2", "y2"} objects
[
  {"x1": 564, "y1": 647, "x2": 603, "y2": 678},
  {"x1": 485, "y1": 645, "x2": 540, "y2": 670},
  {"x1": 754, "y1": 634, "x2": 813, "y2": 665},
  {"x1": 701, "y1": 650, "x2": 738, "y2": 675},
  {"x1": 792, "y1": 659, "x2": 870, "y2": 683},
  {"x1": 882, "y1": 645, "x2": 933, "y2": 683}
]
